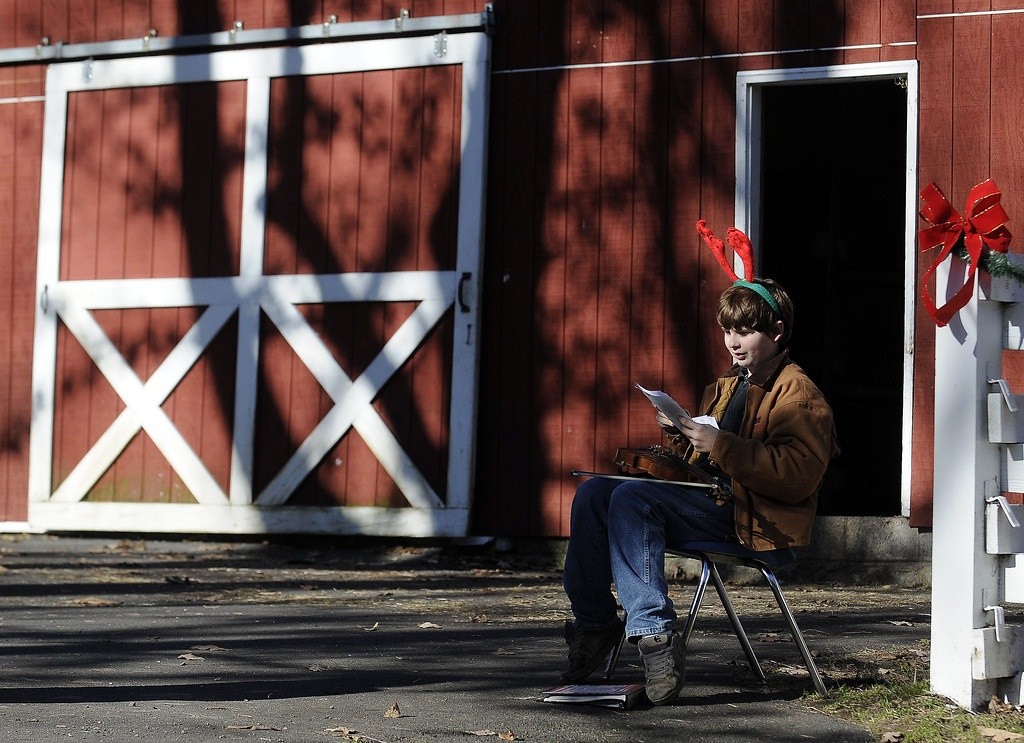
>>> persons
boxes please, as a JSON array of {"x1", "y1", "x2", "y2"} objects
[{"x1": 558, "y1": 278, "x2": 835, "y2": 706}]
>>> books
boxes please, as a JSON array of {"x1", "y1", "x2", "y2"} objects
[{"x1": 539, "y1": 681, "x2": 647, "y2": 711}]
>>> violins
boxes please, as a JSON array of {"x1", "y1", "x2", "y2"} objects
[{"x1": 615, "y1": 442, "x2": 800, "y2": 566}]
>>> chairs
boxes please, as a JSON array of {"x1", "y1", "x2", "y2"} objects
[{"x1": 604, "y1": 545, "x2": 832, "y2": 700}]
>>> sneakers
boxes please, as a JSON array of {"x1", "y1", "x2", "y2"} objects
[
  {"x1": 564, "y1": 617, "x2": 625, "y2": 682},
  {"x1": 638, "y1": 629, "x2": 686, "y2": 705}
]
